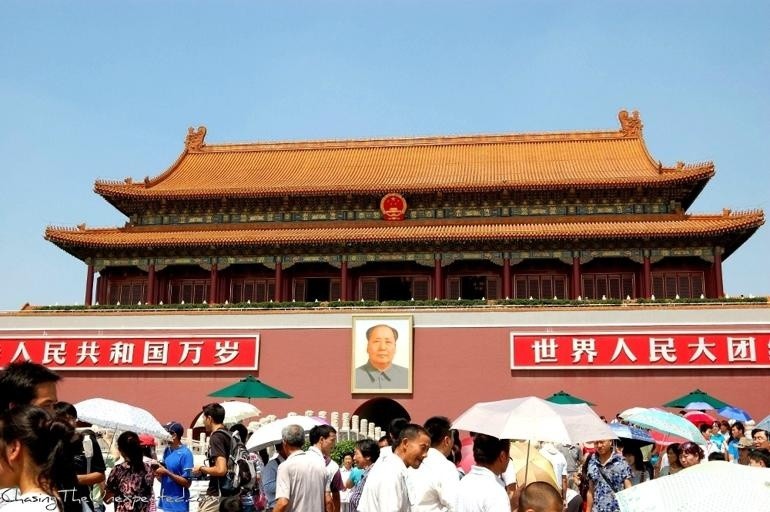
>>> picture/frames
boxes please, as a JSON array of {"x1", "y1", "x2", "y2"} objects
[{"x1": 350, "y1": 313, "x2": 413, "y2": 394}]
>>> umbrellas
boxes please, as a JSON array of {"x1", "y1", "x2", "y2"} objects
[
  {"x1": 71, "y1": 374, "x2": 330, "y2": 450},
  {"x1": 452, "y1": 390, "x2": 769, "y2": 512}
]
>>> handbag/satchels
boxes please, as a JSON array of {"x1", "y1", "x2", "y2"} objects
[{"x1": 89, "y1": 481, "x2": 106, "y2": 505}]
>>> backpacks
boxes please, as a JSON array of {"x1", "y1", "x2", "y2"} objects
[{"x1": 210, "y1": 430, "x2": 256, "y2": 489}]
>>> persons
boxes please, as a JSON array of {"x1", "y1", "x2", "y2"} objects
[
  {"x1": 0, "y1": 362, "x2": 769, "y2": 512},
  {"x1": 354, "y1": 325, "x2": 409, "y2": 390}
]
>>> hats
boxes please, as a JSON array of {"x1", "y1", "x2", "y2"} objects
[
  {"x1": 733, "y1": 437, "x2": 753, "y2": 449},
  {"x1": 163, "y1": 422, "x2": 183, "y2": 436},
  {"x1": 138, "y1": 434, "x2": 158, "y2": 446}
]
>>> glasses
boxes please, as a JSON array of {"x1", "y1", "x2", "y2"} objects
[{"x1": 678, "y1": 443, "x2": 691, "y2": 450}]
